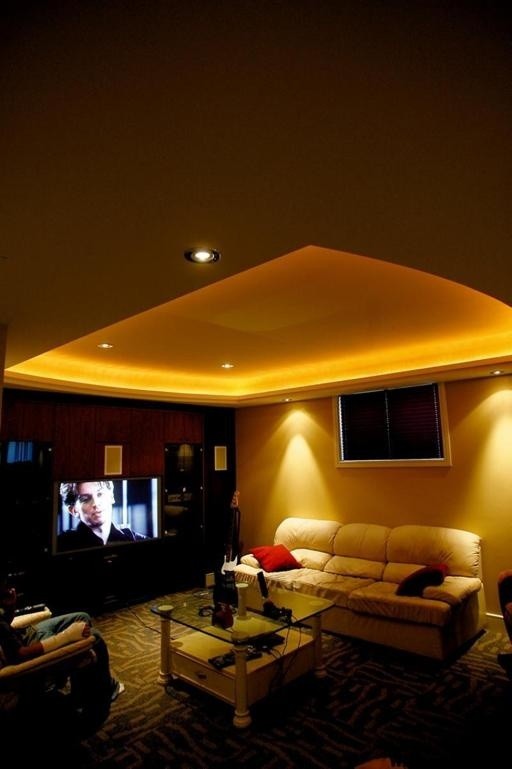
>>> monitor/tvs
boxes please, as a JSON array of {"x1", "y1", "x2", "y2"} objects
[{"x1": 52, "y1": 473, "x2": 164, "y2": 556}]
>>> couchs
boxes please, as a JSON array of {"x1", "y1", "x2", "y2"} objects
[{"x1": 235, "y1": 516, "x2": 488, "y2": 663}]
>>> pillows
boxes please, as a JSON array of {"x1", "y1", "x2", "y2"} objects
[
  {"x1": 248, "y1": 543, "x2": 302, "y2": 572},
  {"x1": 395, "y1": 562, "x2": 449, "y2": 596}
]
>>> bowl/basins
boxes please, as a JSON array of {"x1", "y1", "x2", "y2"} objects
[{"x1": 162, "y1": 505, "x2": 184, "y2": 516}]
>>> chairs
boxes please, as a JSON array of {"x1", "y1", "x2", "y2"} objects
[{"x1": 2, "y1": 630, "x2": 107, "y2": 744}]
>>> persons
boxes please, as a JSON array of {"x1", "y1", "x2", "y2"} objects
[
  {"x1": 1, "y1": 584, "x2": 125, "y2": 701},
  {"x1": 56, "y1": 480, "x2": 151, "y2": 552}
]
